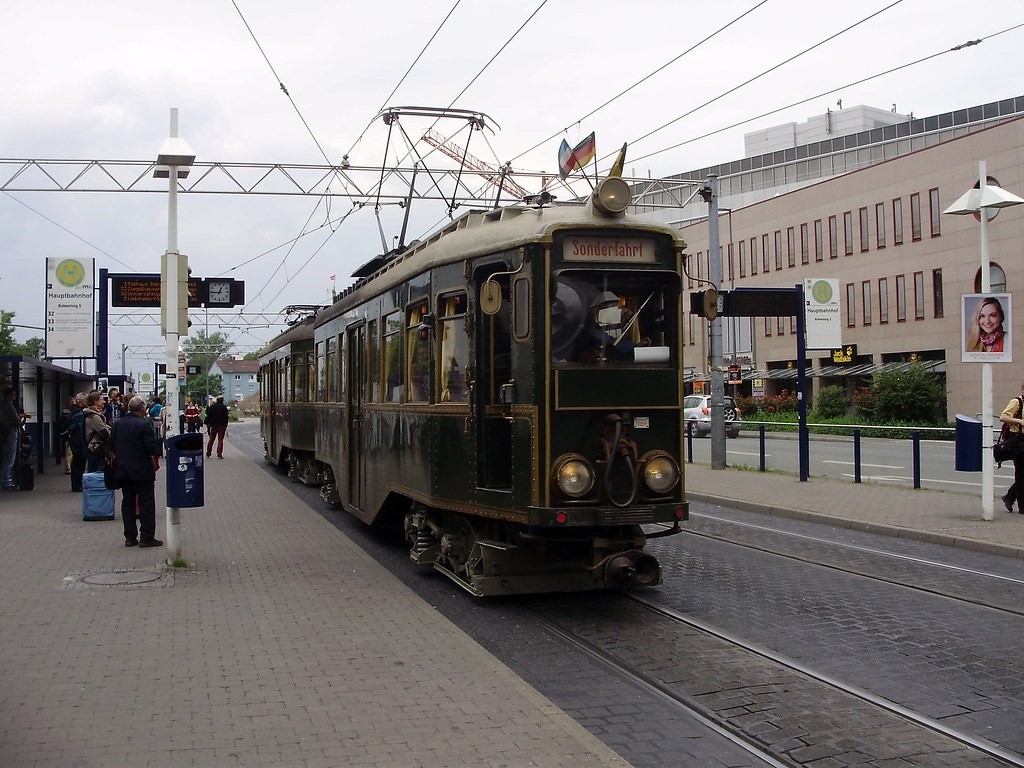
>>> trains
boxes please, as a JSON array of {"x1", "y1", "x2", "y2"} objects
[{"x1": 257, "y1": 175, "x2": 691, "y2": 607}]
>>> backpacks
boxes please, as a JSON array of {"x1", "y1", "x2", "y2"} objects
[
  {"x1": 69, "y1": 412, "x2": 105, "y2": 459},
  {"x1": 61, "y1": 409, "x2": 81, "y2": 443}
]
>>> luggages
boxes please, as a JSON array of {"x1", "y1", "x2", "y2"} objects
[
  {"x1": 81, "y1": 471, "x2": 116, "y2": 521},
  {"x1": 17, "y1": 447, "x2": 34, "y2": 490}
]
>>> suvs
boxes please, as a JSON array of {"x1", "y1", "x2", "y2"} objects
[{"x1": 683, "y1": 396, "x2": 742, "y2": 440}]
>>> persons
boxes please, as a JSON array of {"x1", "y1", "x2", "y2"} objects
[
  {"x1": 183, "y1": 401, "x2": 204, "y2": 433},
  {"x1": 549, "y1": 300, "x2": 652, "y2": 366},
  {"x1": 406, "y1": 342, "x2": 430, "y2": 402},
  {"x1": 999, "y1": 383, "x2": 1024, "y2": 515},
  {"x1": 107, "y1": 395, "x2": 164, "y2": 548},
  {"x1": 61, "y1": 387, "x2": 167, "y2": 518},
  {"x1": 966, "y1": 296, "x2": 1008, "y2": 352},
  {"x1": 204, "y1": 396, "x2": 228, "y2": 459},
  {"x1": 0, "y1": 387, "x2": 24, "y2": 493}
]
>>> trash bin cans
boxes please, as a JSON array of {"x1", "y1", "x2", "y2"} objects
[
  {"x1": 954, "y1": 414, "x2": 983, "y2": 471},
  {"x1": 164, "y1": 432, "x2": 205, "y2": 509}
]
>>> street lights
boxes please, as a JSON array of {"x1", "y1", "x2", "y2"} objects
[
  {"x1": 150, "y1": 105, "x2": 191, "y2": 568},
  {"x1": 938, "y1": 158, "x2": 1024, "y2": 520}
]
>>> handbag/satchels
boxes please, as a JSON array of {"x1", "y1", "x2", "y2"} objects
[
  {"x1": 993, "y1": 442, "x2": 1012, "y2": 462},
  {"x1": 103, "y1": 457, "x2": 122, "y2": 491}
]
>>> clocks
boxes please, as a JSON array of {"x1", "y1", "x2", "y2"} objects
[
  {"x1": 204, "y1": 278, "x2": 235, "y2": 309},
  {"x1": 715, "y1": 292, "x2": 725, "y2": 313}
]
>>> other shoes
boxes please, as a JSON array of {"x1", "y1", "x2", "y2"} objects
[
  {"x1": 3, "y1": 484, "x2": 17, "y2": 491},
  {"x1": 217, "y1": 454, "x2": 223, "y2": 459},
  {"x1": 64, "y1": 468, "x2": 72, "y2": 474},
  {"x1": 206, "y1": 454, "x2": 212, "y2": 459},
  {"x1": 1002, "y1": 495, "x2": 1014, "y2": 513},
  {"x1": 125, "y1": 537, "x2": 139, "y2": 547},
  {"x1": 1018, "y1": 508, "x2": 1024, "y2": 514},
  {"x1": 139, "y1": 538, "x2": 163, "y2": 547}
]
